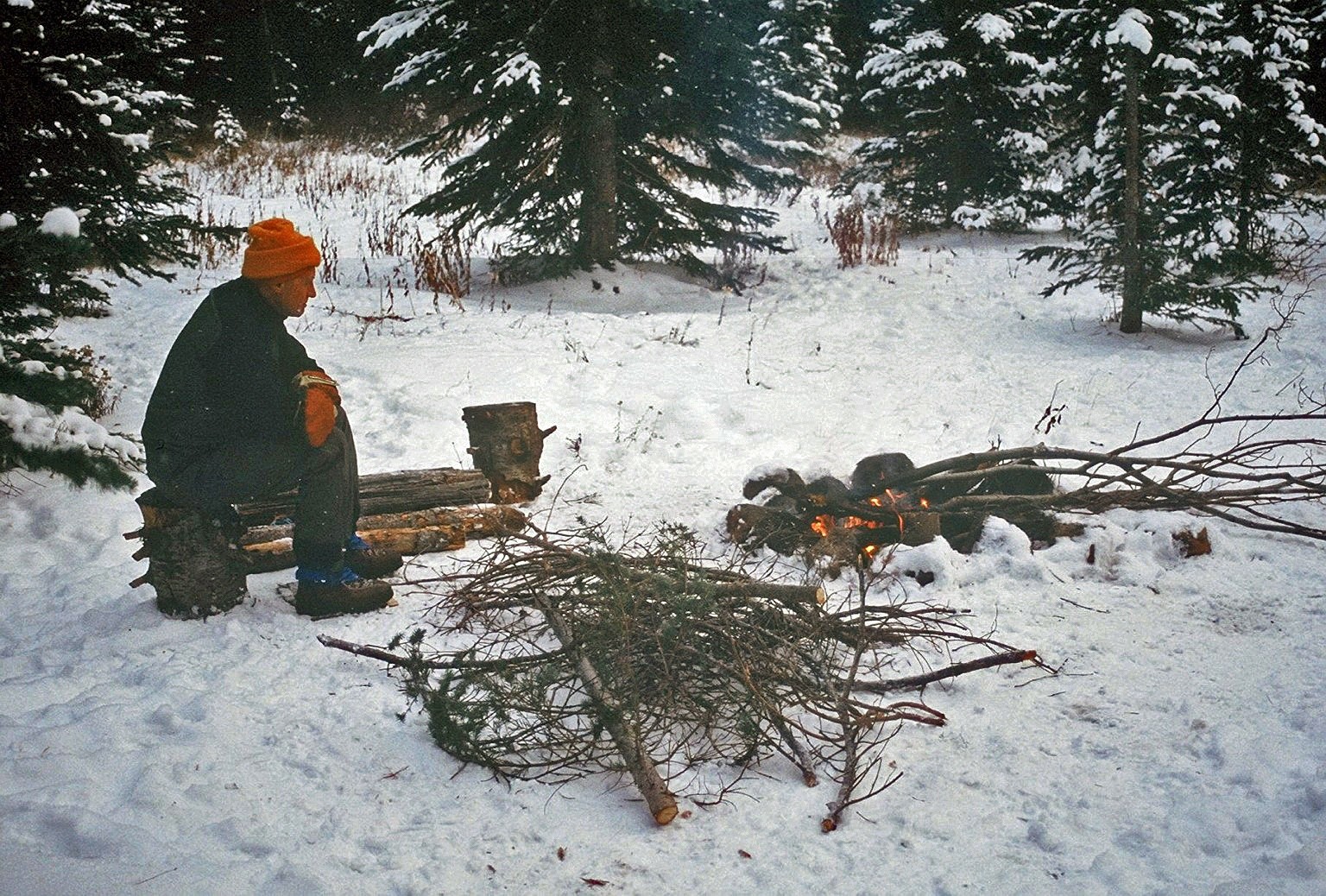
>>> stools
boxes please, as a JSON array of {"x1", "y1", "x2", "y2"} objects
[
  {"x1": 461, "y1": 402, "x2": 557, "y2": 504},
  {"x1": 122, "y1": 487, "x2": 256, "y2": 620}
]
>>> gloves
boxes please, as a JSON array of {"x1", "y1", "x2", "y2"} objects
[{"x1": 294, "y1": 370, "x2": 338, "y2": 449}]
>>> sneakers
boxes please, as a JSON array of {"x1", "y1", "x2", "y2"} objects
[
  {"x1": 296, "y1": 575, "x2": 393, "y2": 616},
  {"x1": 345, "y1": 547, "x2": 403, "y2": 579}
]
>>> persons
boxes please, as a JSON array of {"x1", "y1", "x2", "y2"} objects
[{"x1": 141, "y1": 220, "x2": 404, "y2": 616}]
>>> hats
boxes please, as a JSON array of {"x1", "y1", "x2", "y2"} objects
[{"x1": 242, "y1": 218, "x2": 321, "y2": 284}]
